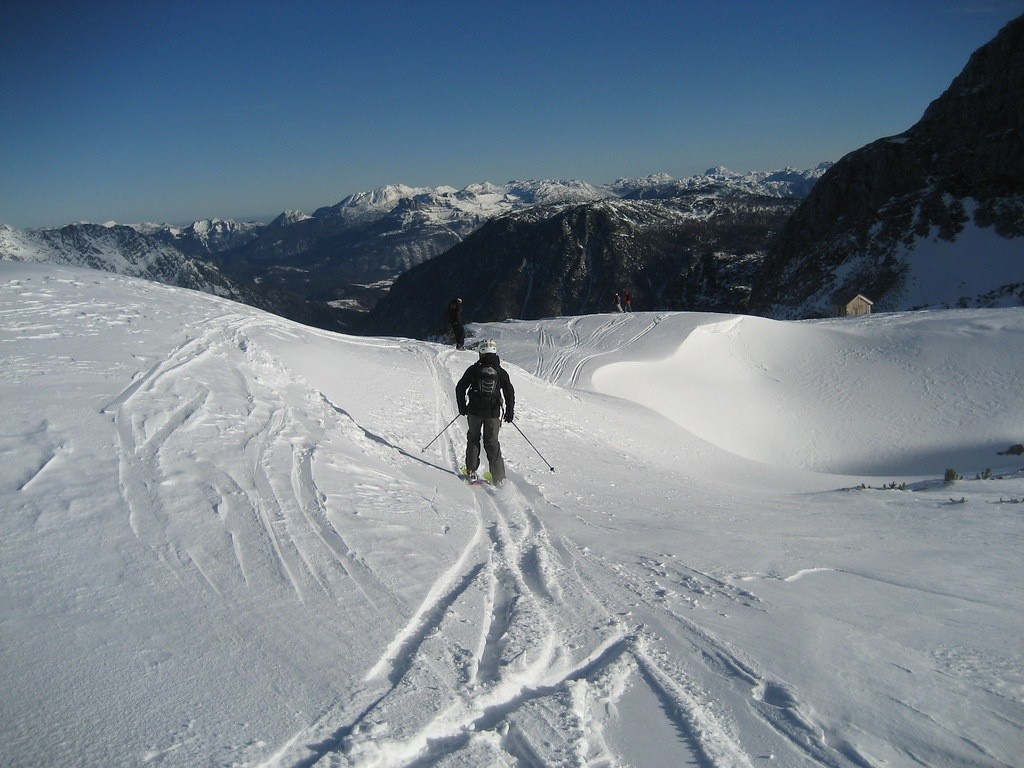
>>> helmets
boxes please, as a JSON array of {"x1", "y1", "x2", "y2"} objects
[{"x1": 478, "y1": 339, "x2": 497, "y2": 354}]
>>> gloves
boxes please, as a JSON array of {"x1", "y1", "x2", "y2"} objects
[
  {"x1": 458, "y1": 402, "x2": 466, "y2": 415},
  {"x1": 504, "y1": 408, "x2": 514, "y2": 423}
]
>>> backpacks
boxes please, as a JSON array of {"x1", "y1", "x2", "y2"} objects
[{"x1": 468, "y1": 361, "x2": 503, "y2": 410}]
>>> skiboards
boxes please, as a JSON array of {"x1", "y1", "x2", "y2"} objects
[{"x1": 458, "y1": 463, "x2": 511, "y2": 494}]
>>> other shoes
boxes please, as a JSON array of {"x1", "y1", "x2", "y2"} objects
[
  {"x1": 457, "y1": 347, "x2": 465, "y2": 351},
  {"x1": 469, "y1": 472, "x2": 478, "y2": 482},
  {"x1": 495, "y1": 480, "x2": 504, "y2": 487}
]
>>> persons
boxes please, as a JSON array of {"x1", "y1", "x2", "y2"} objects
[
  {"x1": 612, "y1": 291, "x2": 624, "y2": 313},
  {"x1": 449, "y1": 295, "x2": 466, "y2": 350},
  {"x1": 455, "y1": 339, "x2": 515, "y2": 489},
  {"x1": 622, "y1": 288, "x2": 632, "y2": 313}
]
